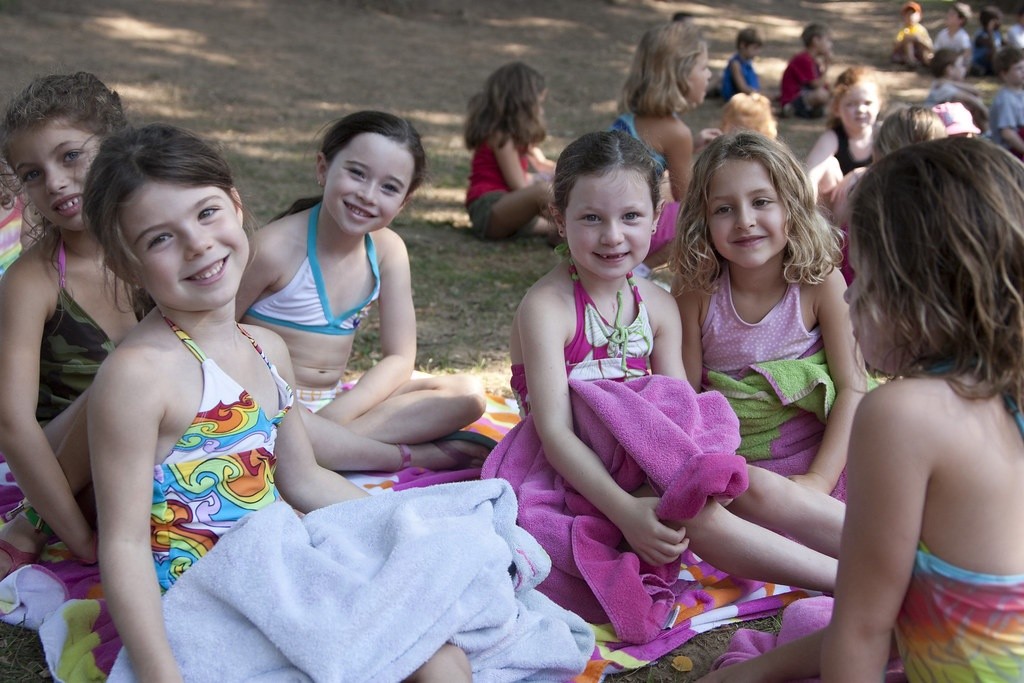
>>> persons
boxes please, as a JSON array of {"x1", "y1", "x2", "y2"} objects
[
  {"x1": 0, "y1": 0, "x2": 1024, "y2": 683},
  {"x1": 234, "y1": 110, "x2": 498, "y2": 473}
]
